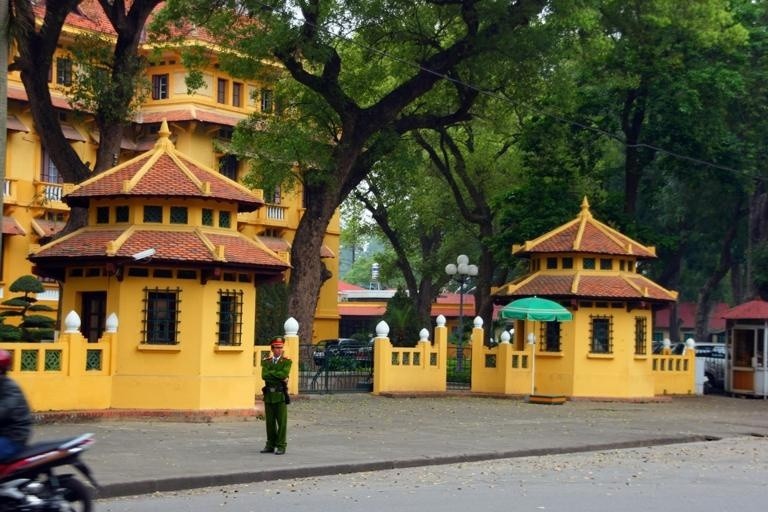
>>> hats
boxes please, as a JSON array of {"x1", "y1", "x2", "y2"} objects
[{"x1": 268, "y1": 336, "x2": 285, "y2": 348}]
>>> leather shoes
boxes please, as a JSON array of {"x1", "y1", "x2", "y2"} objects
[{"x1": 260, "y1": 447, "x2": 285, "y2": 454}]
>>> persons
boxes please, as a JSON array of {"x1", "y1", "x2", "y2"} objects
[
  {"x1": 0, "y1": 351, "x2": 32, "y2": 465},
  {"x1": 260, "y1": 336, "x2": 292, "y2": 455}
]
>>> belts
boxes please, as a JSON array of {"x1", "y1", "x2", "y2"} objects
[{"x1": 265, "y1": 387, "x2": 286, "y2": 392}]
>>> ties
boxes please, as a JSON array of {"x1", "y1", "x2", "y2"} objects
[{"x1": 273, "y1": 358, "x2": 277, "y2": 364}]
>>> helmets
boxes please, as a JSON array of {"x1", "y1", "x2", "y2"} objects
[{"x1": 0, "y1": 350, "x2": 13, "y2": 371}]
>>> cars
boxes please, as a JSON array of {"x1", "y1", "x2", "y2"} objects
[
  {"x1": 313, "y1": 335, "x2": 375, "y2": 367},
  {"x1": 651, "y1": 340, "x2": 726, "y2": 395}
]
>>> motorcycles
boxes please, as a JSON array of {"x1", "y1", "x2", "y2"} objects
[{"x1": 0, "y1": 431, "x2": 103, "y2": 512}]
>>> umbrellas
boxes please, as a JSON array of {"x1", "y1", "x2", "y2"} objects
[{"x1": 498, "y1": 295, "x2": 573, "y2": 395}]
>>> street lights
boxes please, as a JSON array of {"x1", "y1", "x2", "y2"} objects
[{"x1": 445, "y1": 253, "x2": 480, "y2": 372}]
